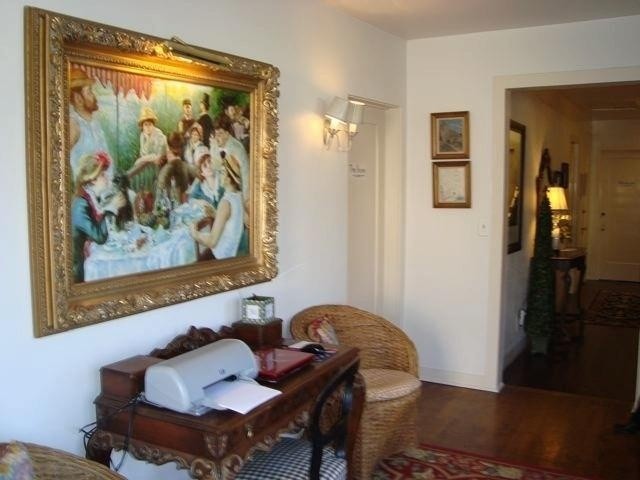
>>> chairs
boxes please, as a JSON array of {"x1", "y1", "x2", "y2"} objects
[
  {"x1": 230, "y1": 354, "x2": 361, "y2": 480},
  {"x1": 291, "y1": 304, "x2": 423, "y2": 480}
]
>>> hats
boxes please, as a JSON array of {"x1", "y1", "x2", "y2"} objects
[
  {"x1": 138, "y1": 106, "x2": 159, "y2": 127},
  {"x1": 70, "y1": 68, "x2": 96, "y2": 88},
  {"x1": 77, "y1": 152, "x2": 104, "y2": 183},
  {"x1": 200, "y1": 93, "x2": 210, "y2": 106},
  {"x1": 220, "y1": 150, "x2": 242, "y2": 186}
]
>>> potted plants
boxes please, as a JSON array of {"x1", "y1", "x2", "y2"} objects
[{"x1": 525, "y1": 195, "x2": 557, "y2": 355}]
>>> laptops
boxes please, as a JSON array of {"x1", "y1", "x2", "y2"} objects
[{"x1": 253, "y1": 346, "x2": 315, "y2": 382}]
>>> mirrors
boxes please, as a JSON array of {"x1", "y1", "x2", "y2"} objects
[{"x1": 509, "y1": 119, "x2": 526, "y2": 255}]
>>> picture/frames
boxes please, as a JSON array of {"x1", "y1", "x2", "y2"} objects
[
  {"x1": 430, "y1": 110, "x2": 472, "y2": 209},
  {"x1": 23, "y1": 5, "x2": 280, "y2": 339}
]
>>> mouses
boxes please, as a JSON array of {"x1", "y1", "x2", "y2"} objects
[{"x1": 301, "y1": 342, "x2": 329, "y2": 353}]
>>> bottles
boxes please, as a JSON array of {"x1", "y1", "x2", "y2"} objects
[{"x1": 153, "y1": 176, "x2": 180, "y2": 215}]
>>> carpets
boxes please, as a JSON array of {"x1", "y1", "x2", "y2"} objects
[
  {"x1": 580, "y1": 285, "x2": 639, "y2": 328},
  {"x1": 371, "y1": 441, "x2": 598, "y2": 480}
]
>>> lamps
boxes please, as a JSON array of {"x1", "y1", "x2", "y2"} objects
[
  {"x1": 545, "y1": 186, "x2": 569, "y2": 251},
  {"x1": 322, "y1": 97, "x2": 366, "y2": 153}
]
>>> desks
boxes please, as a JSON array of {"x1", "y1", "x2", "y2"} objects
[{"x1": 85, "y1": 338, "x2": 365, "y2": 480}]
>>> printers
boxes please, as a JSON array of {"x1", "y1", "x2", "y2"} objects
[{"x1": 141, "y1": 335, "x2": 259, "y2": 415}]
